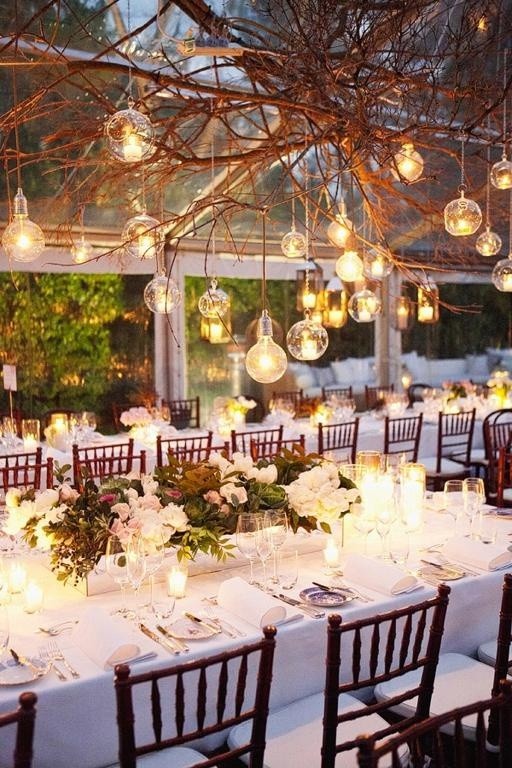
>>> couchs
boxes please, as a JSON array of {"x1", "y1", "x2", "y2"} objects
[{"x1": 297, "y1": 359, "x2": 470, "y2": 411}]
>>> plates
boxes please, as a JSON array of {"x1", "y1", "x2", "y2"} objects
[
  {"x1": 298, "y1": 587, "x2": 353, "y2": 606},
  {"x1": 0, "y1": 656, "x2": 51, "y2": 686},
  {"x1": 163, "y1": 617, "x2": 219, "y2": 640}
]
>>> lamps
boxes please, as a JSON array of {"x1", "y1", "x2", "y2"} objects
[
  {"x1": 197, "y1": 53, "x2": 231, "y2": 318},
  {"x1": 286, "y1": 116, "x2": 329, "y2": 360},
  {"x1": 390, "y1": 295, "x2": 411, "y2": 331},
  {"x1": 491, "y1": 48, "x2": 512, "y2": 189},
  {"x1": 390, "y1": 139, "x2": 424, "y2": 181},
  {"x1": 201, "y1": 302, "x2": 233, "y2": 345},
  {"x1": 281, "y1": 180, "x2": 306, "y2": 258},
  {"x1": 104, "y1": 0, "x2": 155, "y2": 163},
  {"x1": 476, "y1": 144, "x2": 502, "y2": 255},
  {"x1": 322, "y1": 276, "x2": 349, "y2": 328},
  {"x1": 417, "y1": 233, "x2": 439, "y2": 323},
  {"x1": 492, "y1": 188, "x2": 512, "y2": 292},
  {"x1": 144, "y1": 184, "x2": 181, "y2": 314},
  {"x1": 1, "y1": 66, "x2": 45, "y2": 262},
  {"x1": 336, "y1": 248, "x2": 364, "y2": 282},
  {"x1": 444, "y1": 135, "x2": 482, "y2": 236},
  {"x1": 363, "y1": 197, "x2": 395, "y2": 281},
  {"x1": 119, "y1": 161, "x2": 166, "y2": 259},
  {"x1": 347, "y1": 281, "x2": 383, "y2": 323},
  {"x1": 296, "y1": 268, "x2": 324, "y2": 312},
  {"x1": 245, "y1": 214, "x2": 288, "y2": 383},
  {"x1": 327, "y1": 173, "x2": 356, "y2": 248},
  {"x1": 71, "y1": 227, "x2": 94, "y2": 264}
]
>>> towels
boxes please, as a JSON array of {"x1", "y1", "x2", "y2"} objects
[
  {"x1": 69, "y1": 611, "x2": 161, "y2": 673},
  {"x1": 443, "y1": 533, "x2": 511, "y2": 573},
  {"x1": 344, "y1": 556, "x2": 424, "y2": 596},
  {"x1": 216, "y1": 578, "x2": 303, "y2": 631}
]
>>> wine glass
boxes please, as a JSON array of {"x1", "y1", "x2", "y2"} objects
[
  {"x1": 236, "y1": 514, "x2": 265, "y2": 591},
  {"x1": 387, "y1": 453, "x2": 407, "y2": 516},
  {"x1": 443, "y1": 480, "x2": 468, "y2": 543},
  {"x1": 388, "y1": 395, "x2": 410, "y2": 416},
  {"x1": 138, "y1": 533, "x2": 164, "y2": 609},
  {"x1": 82, "y1": 411, "x2": 96, "y2": 445},
  {"x1": 462, "y1": 477, "x2": 485, "y2": 541},
  {"x1": 432, "y1": 389, "x2": 443, "y2": 412},
  {"x1": 352, "y1": 505, "x2": 376, "y2": 558},
  {"x1": 105, "y1": 534, "x2": 136, "y2": 620},
  {"x1": 377, "y1": 495, "x2": 397, "y2": 560},
  {"x1": 255, "y1": 521, "x2": 274, "y2": 594},
  {"x1": 389, "y1": 531, "x2": 410, "y2": 567},
  {"x1": 0, "y1": 417, "x2": 19, "y2": 454},
  {"x1": 263, "y1": 508, "x2": 288, "y2": 583},
  {"x1": 150, "y1": 407, "x2": 171, "y2": 440},
  {"x1": 421, "y1": 388, "x2": 433, "y2": 411},
  {"x1": 70, "y1": 413, "x2": 83, "y2": 447},
  {"x1": 126, "y1": 559, "x2": 147, "y2": 625},
  {"x1": 268, "y1": 399, "x2": 297, "y2": 415},
  {"x1": 377, "y1": 523, "x2": 391, "y2": 561},
  {"x1": 332, "y1": 395, "x2": 356, "y2": 421}
]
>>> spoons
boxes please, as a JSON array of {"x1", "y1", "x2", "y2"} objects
[{"x1": 48, "y1": 626, "x2": 73, "y2": 635}]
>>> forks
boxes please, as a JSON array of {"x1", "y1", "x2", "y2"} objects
[
  {"x1": 332, "y1": 576, "x2": 375, "y2": 601},
  {"x1": 47, "y1": 641, "x2": 80, "y2": 679},
  {"x1": 39, "y1": 620, "x2": 73, "y2": 633},
  {"x1": 38, "y1": 647, "x2": 67, "y2": 682},
  {"x1": 155, "y1": 624, "x2": 190, "y2": 652},
  {"x1": 207, "y1": 606, "x2": 247, "y2": 637}
]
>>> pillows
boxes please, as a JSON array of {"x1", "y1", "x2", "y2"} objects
[
  {"x1": 313, "y1": 365, "x2": 335, "y2": 386},
  {"x1": 330, "y1": 359, "x2": 358, "y2": 385},
  {"x1": 486, "y1": 348, "x2": 512, "y2": 373},
  {"x1": 363, "y1": 357, "x2": 378, "y2": 382},
  {"x1": 404, "y1": 355, "x2": 431, "y2": 383},
  {"x1": 290, "y1": 362, "x2": 319, "y2": 389},
  {"x1": 465, "y1": 353, "x2": 489, "y2": 376},
  {"x1": 401, "y1": 349, "x2": 418, "y2": 366},
  {"x1": 347, "y1": 356, "x2": 369, "y2": 383}
]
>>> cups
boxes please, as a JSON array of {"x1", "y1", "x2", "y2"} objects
[
  {"x1": 51, "y1": 414, "x2": 69, "y2": 447},
  {"x1": 322, "y1": 546, "x2": 344, "y2": 576},
  {"x1": 355, "y1": 450, "x2": 379, "y2": 486},
  {"x1": 164, "y1": 565, "x2": 189, "y2": 598},
  {"x1": 22, "y1": 419, "x2": 40, "y2": 458},
  {"x1": 0, "y1": 604, "x2": 10, "y2": 663},
  {"x1": 478, "y1": 509, "x2": 497, "y2": 545},
  {"x1": 400, "y1": 463, "x2": 427, "y2": 530}
]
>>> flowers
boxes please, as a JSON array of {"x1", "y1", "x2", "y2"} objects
[
  {"x1": 120, "y1": 405, "x2": 152, "y2": 426},
  {"x1": 487, "y1": 370, "x2": 512, "y2": 396},
  {"x1": 211, "y1": 395, "x2": 257, "y2": 425},
  {"x1": 0, "y1": 443, "x2": 363, "y2": 585},
  {"x1": 442, "y1": 378, "x2": 484, "y2": 408}
]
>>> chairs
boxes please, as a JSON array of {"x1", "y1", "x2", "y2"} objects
[
  {"x1": 35, "y1": 408, "x2": 77, "y2": 442},
  {"x1": 0, "y1": 408, "x2": 23, "y2": 439},
  {"x1": 227, "y1": 583, "x2": 451, "y2": 768},
  {"x1": 156, "y1": 430, "x2": 213, "y2": 466},
  {"x1": 72, "y1": 437, "x2": 134, "y2": 489},
  {"x1": 365, "y1": 382, "x2": 395, "y2": 411},
  {"x1": 357, "y1": 677, "x2": 512, "y2": 768},
  {"x1": 319, "y1": 416, "x2": 360, "y2": 480},
  {"x1": 451, "y1": 408, "x2": 512, "y2": 504},
  {"x1": 109, "y1": 398, "x2": 146, "y2": 435},
  {"x1": 0, "y1": 691, "x2": 38, "y2": 767},
  {"x1": 494, "y1": 408, "x2": 512, "y2": 501},
  {"x1": 272, "y1": 387, "x2": 303, "y2": 418},
  {"x1": 408, "y1": 382, "x2": 434, "y2": 408},
  {"x1": 231, "y1": 424, "x2": 284, "y2": 458},
  {"x1": 233, "y1": 394, "x2": 266, "y2": 423},
  {"x1": 322, "y1": 384, "x2": 352, "y2": 406},
  {"x1": 477, "y1": 637, "x2": 512, "y2": 675},
  {"x1": 418, "y1": 407, "x2": 477, "y2": 492},
  {"x1": 168, "y1": 440, "x2": 229, "y2": 464},
  {"x1": 384, "y1": 411, "x2": 424, "y2": 471},
  {"x1": 0, "y1": 446, "x2": 42, "y2": 488},
  {"x1": 101, "y1": 624, "x2": 277, "y2": 767},
  {"x1": 496, "y1": 445, "x2": 512, "y2": 507},
  {"x1": 250, "y1": 434, "x2": 305, "y2": 462},
  {"x1": 372, "y1": 573, "x2": 512, "y2": 768},
  {"x1": 161, "y1": 395, "x2": 200, "y2": 430},
  {"x1": 73, "y1": 449, "x2": 146, "y2": 492},
  {"x1": 0, "y1": 456, "x2": 53, "y2": 504}
]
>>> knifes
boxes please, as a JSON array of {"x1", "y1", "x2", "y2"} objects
[
  {"x1": 184, "y1": 612, "x2": 222, "y2": 634},
  {"x1": 202, "y1": 617, "x2": 236, "y2": 639},
  {"x1": 271, "y1": 594, "x2": 325, "y2": 619},
  {"x1": 138, "y1": 622, "x2": 180, "y2": 655},
  {"x1": 9, "y1": 649, "x2": 44, "y2": 677},
  {"x1": 311, "y1": 581, "x2": 360, "y2": 599}
]
